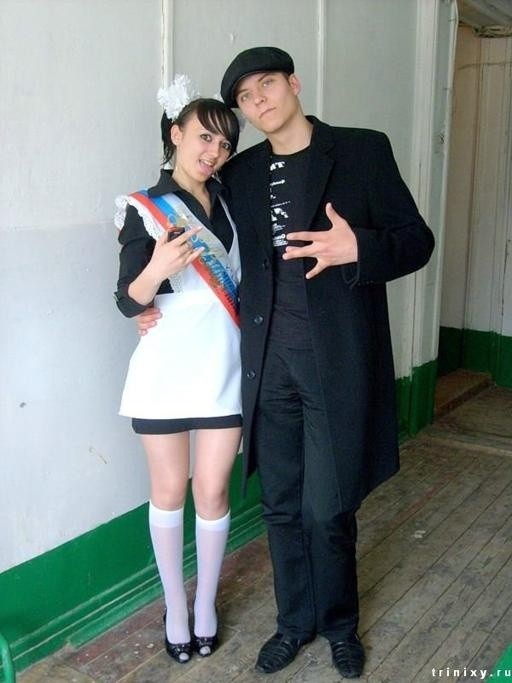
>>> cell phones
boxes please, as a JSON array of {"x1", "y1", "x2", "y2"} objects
[{"x1": 167, "y1": 225, "x2": 186, "y2": 246}]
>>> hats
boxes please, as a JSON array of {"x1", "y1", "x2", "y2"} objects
[{"x1": 221, "y1": 46, "x2": 294, "y2": 108}]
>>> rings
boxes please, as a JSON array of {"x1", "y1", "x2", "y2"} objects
[{"x1": 183, "y1": 241, "x2": 193, "y2": 249}]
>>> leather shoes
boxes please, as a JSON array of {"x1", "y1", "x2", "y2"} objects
[
  {"x1": 255, "y1": 631, "x2": 310, "y2": 674},
  {"x1": 329, "y1": 633, "x2": 366, "y2": 679},
  {"x1": 163, "y1": 610, "x2": 190, "y2": 663},
  {"x1": 193, "y1": 598, "x2": 219, "y2": 657}
]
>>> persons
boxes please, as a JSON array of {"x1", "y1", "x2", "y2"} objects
[
  {"x1": 113, "y1": 73, "x2": 244, "y2": 663},
  {"x1": 134, "y1": 45, "x2": 435, "y2": 675}
]
